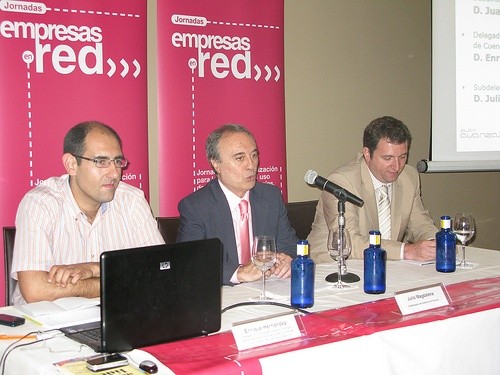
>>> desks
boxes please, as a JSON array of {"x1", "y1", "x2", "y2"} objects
[{"x1": 0, "y1": 245, "x2": 500, "y2": 375}]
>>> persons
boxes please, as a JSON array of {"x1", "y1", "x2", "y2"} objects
[
  {"x1": 306, "y1": 116, "x2": 439, "y2": 261},
  {"x1": 176, "y1": 124, "x2": 299, "y2": 285},
  {"x1": 11, "y1": 121, "x2": 166, "y2": 305}
]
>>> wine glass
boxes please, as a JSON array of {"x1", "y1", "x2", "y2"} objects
[
  {"x1": 327, "y1": 228, "x2": 352, "y2": 289},
  {"x1": 251, "y1": 236, "x2": 277, "y2": 300},
  {"x1": 454, "y1": 212, "x2": 478, "y2": 270}
]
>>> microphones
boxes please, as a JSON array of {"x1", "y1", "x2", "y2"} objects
[{"x1": 304, "y1": 170, "x2": 365, "y2": 208}]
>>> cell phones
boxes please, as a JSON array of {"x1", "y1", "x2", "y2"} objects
[
  {"x1": 86, "y1": 353, "x2": 129, "y2": 371},
  {"x1": 0, "y1": 313, "x2": 25, "y2": 327}
]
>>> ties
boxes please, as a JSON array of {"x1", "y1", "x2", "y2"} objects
[
  {"x1": 377, "y1": 186, "x2": 391, "y2": 240},
  {"x1": 239, "y1": 200, "x2": 251, "y2": 266}
]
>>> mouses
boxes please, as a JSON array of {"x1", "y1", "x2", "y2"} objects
[{"x1": 139, "y1": 360, "x2": 158, "y2": 374}]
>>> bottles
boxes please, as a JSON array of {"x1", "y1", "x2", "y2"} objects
[
  {"x1": 435, "y1": 216, "x2": 457, "y2": 273},
  {"x1": 291, "y1": 239, "x2": 314, "y2": 308},
  {"x1": 363, "y1": 231, "x2": 386, "y2": 294}
]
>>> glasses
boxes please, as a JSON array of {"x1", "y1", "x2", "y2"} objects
[{"x1": 73, "y1": 157, "x2": 128, "y2": 168}]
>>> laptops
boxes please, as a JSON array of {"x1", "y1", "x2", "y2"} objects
[{"x1": 59, "y1": 238, "x2": 224, "y2": 354}]
